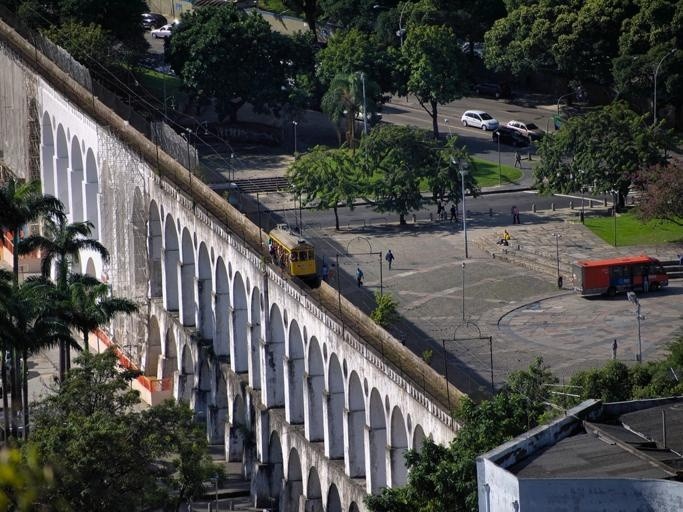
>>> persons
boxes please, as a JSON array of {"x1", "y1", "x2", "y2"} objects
[
  {"x1": 354, "y1": 267, "x2": 364, "y2": 290},
  {"x1": 383, "y1": 247, "x2": 394, "y2": 274},
  {"x1": 405, "y1": 201, "x2": 524, "y2": 226},
  {"x1": 264, "y1": 242, "x2": 339, "y2": 284},
  {"x1": 642, "y1": 274, "x2": 649, "y2": 297},
  {"x1": 610, "y1": 339, "x2": 618, "y2": 362},
  {"x1": 513, "y1": 150, "x2": 523, "y2": 169},
  {"x1": 496, "y1": 229, "x2": 511, "y2": 246}
]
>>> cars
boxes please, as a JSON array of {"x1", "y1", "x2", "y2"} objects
[
  {"x1": 342, "y1": 105, "x2": 384, "y2": 122},
  {"x1": 139, "y1": 11, "x2": 168, "y2": 32},
  {"x1": 461, "y1": 41, "x2": 486, "y2": 58},
  {"x1": 150, "y1": 24, "x2": 178, "y2": 39},
  {"x1": 0, "y1": 350, "x2": 25, "y2": 397},
  {"x1": 459, "y1": 109, "x2": 499, "y2": 131},
  {"x1": 506, "y1": 118, "x2": 546, "y2": 142},
  {"x1": 491, "y1": 125, "x2": 531, "y2": 147}
]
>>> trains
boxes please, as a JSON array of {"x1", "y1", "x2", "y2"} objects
[{"x1": 265, "y1": 220, "x2": 318, "y2": 278}]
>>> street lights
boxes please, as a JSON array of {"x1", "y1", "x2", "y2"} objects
[
  {"x1": 444, "y1": 118, "x2": 452, "y2": 137},
  {"x1": 353, "y1": 69, "x2": 368, "y2": 136},
  {"x1": 227, "y1": 151, "x2": 240, "y2": 202},
  {"x1": 179, "y1": 132, "x2": 192, "y2": 186},
  {"x1": 162, "y1": 94, "x2": 178, "y2": 126},
  {"x1": 460, "y1": 260, "x2": 466, "y2": 322},
  {"x1": 549, "y1": 231, "x2": 564, "y2": 289},
  {"x1": 579, "y1": 187, "x2": 586, "y2": 214},
  {"x1": 192, "y1": 119, "x2": 210, "y2": 149},
  {"x1": 372, "y1": 1, "x2": 407, "y2": 48},
  {"x1": 448, "y1": 156, "x2": 471, "y2": 258},
  {"x1": 653, "y1": 48, "x2": 678, "y2": 127},
  {"x1": 626, "y1": 289, "x2": 643, "y2": 364},
  {"x1": 291, "y1": 120, "x2": 299, "y2": 216}
]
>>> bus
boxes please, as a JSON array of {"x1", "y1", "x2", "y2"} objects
[{"x1": 569, "y1": 256, "x2": 671, "y2": 300}]
